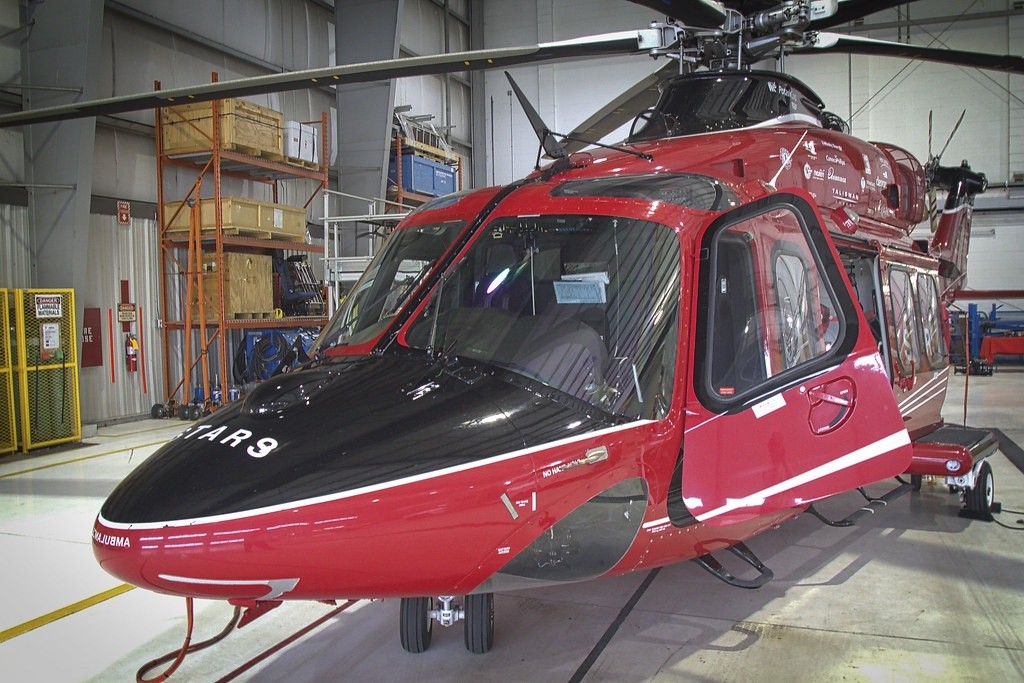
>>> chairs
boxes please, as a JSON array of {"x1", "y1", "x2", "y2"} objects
[{"x1": 473, "y1": 242, "x2": 654, "y2": 332}]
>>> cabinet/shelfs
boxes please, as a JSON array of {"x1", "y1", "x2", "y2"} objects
[
  {"x1": 388, "y1": 136, "x2": 463, "y2": 213},
  {"x1": 319, "y1": 189, "x2": 429, "y2": 282},
  {"x1": 154, "y1": 71, "x2": 329, "y2": 407}
]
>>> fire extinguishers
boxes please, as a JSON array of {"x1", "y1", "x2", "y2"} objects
[{"x1": 126, "y1": 333, "x2": 138, "y2": 372}]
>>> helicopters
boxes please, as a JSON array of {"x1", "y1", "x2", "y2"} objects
[{"x1": 0, "y1": 1, "x2": 1024, "y2": 681}]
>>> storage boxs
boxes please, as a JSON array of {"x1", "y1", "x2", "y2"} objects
[
  {"x1": 283, "y1": 120, "x2": 319, "y2": 165},
  {"x1": 161, "y1": 98, "x2": 283, "y2": 161},
  {"x1": 164, "y1": 196, "x2": 306, "y2": 244},
  {"x1": 191, "y1": 252, "x2": 274, "y2": 320},
  {"x1": 388, "y1": 153, "x2": 456, "y2": 197}
]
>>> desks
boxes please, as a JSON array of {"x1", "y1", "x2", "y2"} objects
[{"x1": 981, "y1": 336, "x2": 1024, "y2": 374}]
